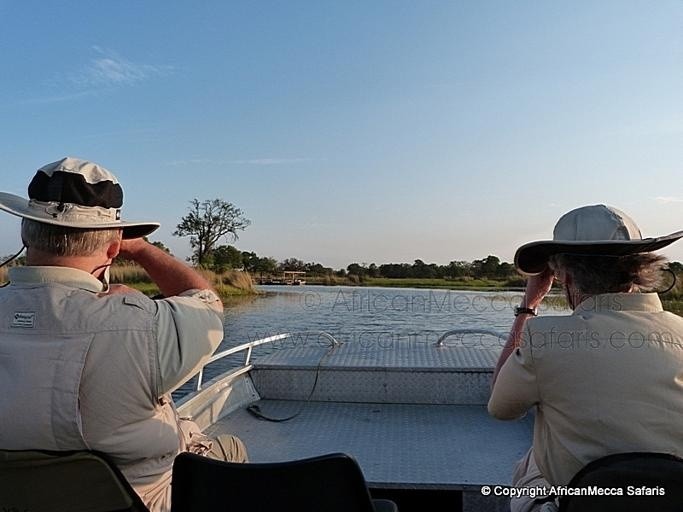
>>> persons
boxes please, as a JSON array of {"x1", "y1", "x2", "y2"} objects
[
  {"x1": 0, "y1": 157, "x2": 248, "y2": 512},
  {"x1": 487, "y1": 204, "x2": 683, "y2": 512}
]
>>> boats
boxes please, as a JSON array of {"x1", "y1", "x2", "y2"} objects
[{"x1": 268, "y1": 270, "x2": 306, "y2": 285}]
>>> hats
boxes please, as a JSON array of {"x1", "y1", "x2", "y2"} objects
[
  {"x1": 514, "y1": 204, "x2": 683, "y2": 273},
  {"x1": 0, "y1": 156, "x2": 161, "y2": 240}
]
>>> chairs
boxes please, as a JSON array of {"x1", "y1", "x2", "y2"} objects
[{"x1": 0, "y1": 448, "x2": 682, "y2": 511}]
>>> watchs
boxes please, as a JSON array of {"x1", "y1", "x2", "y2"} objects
[{"x1": 514, "y1": 303, "x2": 538, "y2": 316}]
